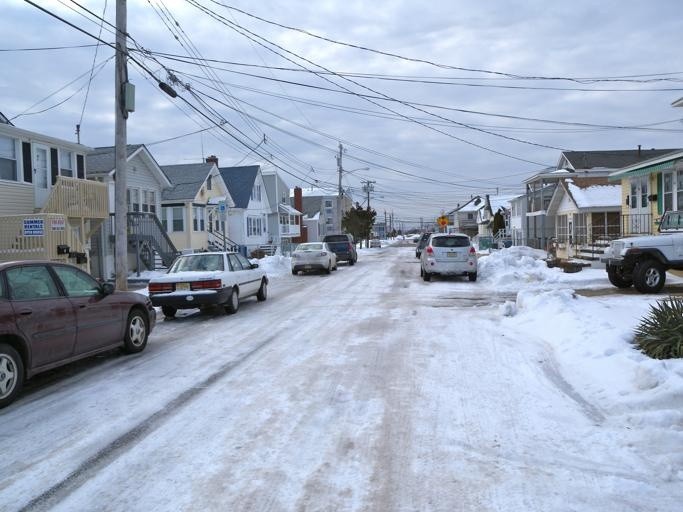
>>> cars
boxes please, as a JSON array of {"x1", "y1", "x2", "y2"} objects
[
  {"x1": 291, "y1": 234, "x2": 357, "y2": 275},
  {"x1": 414, "y1": 232, "x2": 477, "y2": 281},
  {"x1": 149, "y1": 250, "x2": 269, "y2": 318},
  {"x1": 370, "y1": 239, "x2": 381, "y2": 248},
  {"x1": 0, "y1": 259, "x2": 157, "y2": 409}
]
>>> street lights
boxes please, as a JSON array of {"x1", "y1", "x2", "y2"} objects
[
  {"x1": 367, "y1": 196, "x2": 384, "y2": 248},
  {"x1": 337, "y1": 167, "x2": 369, "y2": 234}
]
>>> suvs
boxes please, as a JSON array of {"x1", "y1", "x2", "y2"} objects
[{"x1": 601, "y1": 208, "x2": 683, "y2": 293}]
